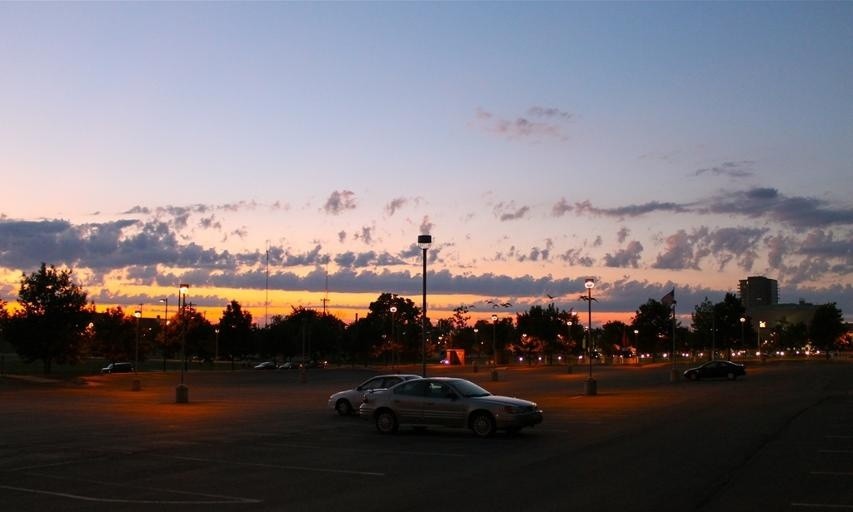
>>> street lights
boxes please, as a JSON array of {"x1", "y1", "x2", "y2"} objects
[
  {"x1": 390, "y1": 307, "x2": 397, "y2": 341},
  {"x1": 160, "y1": 298, "x2": 167, "y2": 371},
  {"x1": 491, "y1": 314, "x2": 498, "y2": 380},
  {"x1": 584, "y1": 279, "x2": 597, "y2": 395},
  {"x1": 418, "y1": 235, "x2": 431, "y2": 375},
  {"x1": 176, "y1": 284, "x2": 188, "y2": 403}
]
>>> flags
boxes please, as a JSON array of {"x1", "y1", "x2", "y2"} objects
[{"x1": 661, "y1": 290, "x2": 674, "y2": 309}]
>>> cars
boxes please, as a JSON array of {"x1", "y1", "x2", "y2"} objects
[
  {"x1": 101, "y1": 361, "x2": 135, "y2": 374},
  {"x1": 682, "y1": 359, "x2": 746, "y2": 381},
  {"x1": 255, "y1": 361, "x2": 295, "y2": 369},
  {"x1": 328, "y1": 373, "x2": 544, "y2": 439}
]
